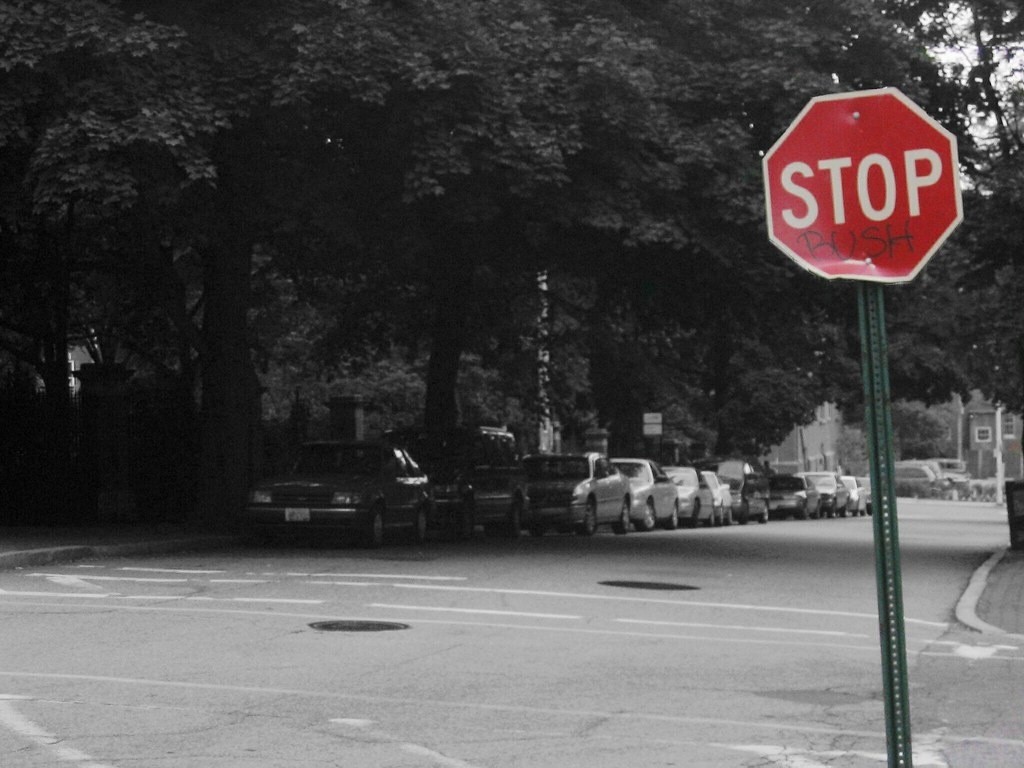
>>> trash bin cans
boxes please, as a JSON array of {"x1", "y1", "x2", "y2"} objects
[{"x1": 1005, "y1": 478, "x2": 1024, "y2": 550}]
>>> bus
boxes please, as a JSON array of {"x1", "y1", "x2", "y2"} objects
[{"x1": 897, "y1": 459, "x2": 971, "y2": 501}]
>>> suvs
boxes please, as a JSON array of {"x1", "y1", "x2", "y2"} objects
[
  {"x1": 690, "y1": 456, "x2": 772, "y2": 525},
  {"x1": 380, "y1": 423, "x2": 529, "y2": 539}
]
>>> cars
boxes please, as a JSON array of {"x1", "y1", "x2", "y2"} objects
[
  {"x1": 608, "y1": 457, "x2": 684, "y2": 532},
  {"x1": 698, "y1": 472, "x2": 735, "y2": 528},
  {"x1": 247, "y1": 440, "x2": 434, "y2": 545},
  {"x1": 843, "y1": 475, "x2": 873, "y2": 521},
  {"x1": 767, "y1": 473, "x2": 822, "y2": 523},
  {"x1": 802, "y1": 472, "x2": 851, "y2": 521},
  {"x1": 659, "y1": 465, "x2": 703, "y2": 529},
  {"x1": 521, "y1": 450, "x2": 634, "y2": 537}
]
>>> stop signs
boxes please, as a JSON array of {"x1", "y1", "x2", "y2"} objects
[{"x1": 762, "y1": 85, "x2": 963, "y2": 284}]
